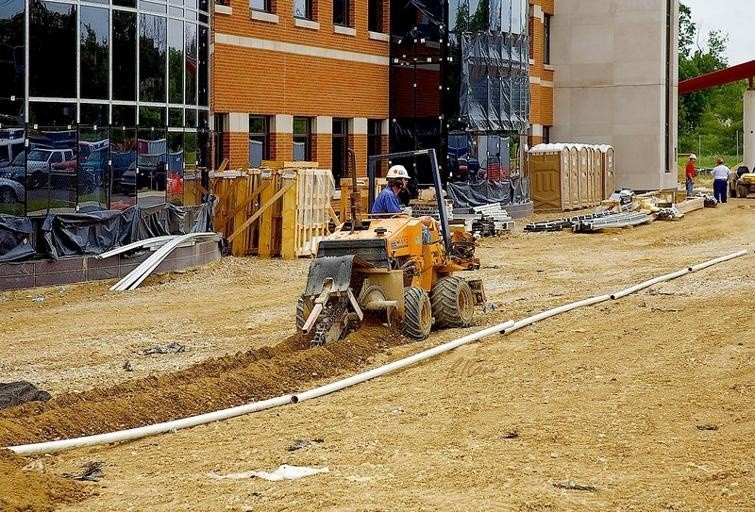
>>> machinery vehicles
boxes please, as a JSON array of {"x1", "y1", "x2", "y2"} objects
[
  {"x1": 729, "y1": 165, "x2": 755, "y2": 198},
  {"x1": 295, "y1": 147, "x2": 487, "y2": 347}
]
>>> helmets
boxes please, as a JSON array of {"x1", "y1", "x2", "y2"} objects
[
  {"x1": 386, "y1": 164, "x2": 412, "y2": 179},
  {"x1": 689, "y1": 154, "x2": 696, "y2": 159},
  {"x1": 717, "y1": 157, "x2": 724, "y2": 163}
]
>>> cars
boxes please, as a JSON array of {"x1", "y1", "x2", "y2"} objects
[
  {"x1": 446, "y1": 153, "x2": 511, "y2": 180},
  {"x1": 0, "y1": 122, "x2": 176, "y2": 203}
]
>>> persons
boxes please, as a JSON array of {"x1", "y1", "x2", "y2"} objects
[
  {"x1": 710, "y1": 158, "x2": 732, "y2": 203},
  {"x1": 372, "y1": 164, "x2": 432, "y2": 244},
  {"x1": 686, "y1": 153, "x2": 697, "y2": 195}
]
